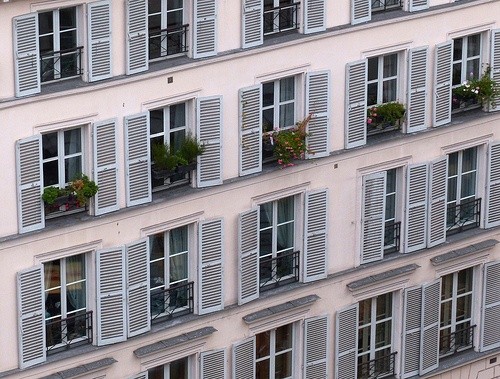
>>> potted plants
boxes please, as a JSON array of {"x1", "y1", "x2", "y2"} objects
[
  {"x1": 151, "y1": 141, "x2": 179, "y2": 181},
  {"x1": 68, "y1": 172, "x2": 98, "y2": 209},
  {"x1": 171, "y1": 131, "x2": 209, "y2": 177},
  {"x1": 41, "y1": 186, "x2": 70, "y2": 211}
]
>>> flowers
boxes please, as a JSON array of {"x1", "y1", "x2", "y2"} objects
[
  {"x1": 453, "y1": 66, "x2": 500, "y2": 110},
  {"x1": 367, "y1": 102, "x2": 406, "y2": 132},
  {"x1": 263, "y1": 113, "x2": 317, "y2": 168}
]
